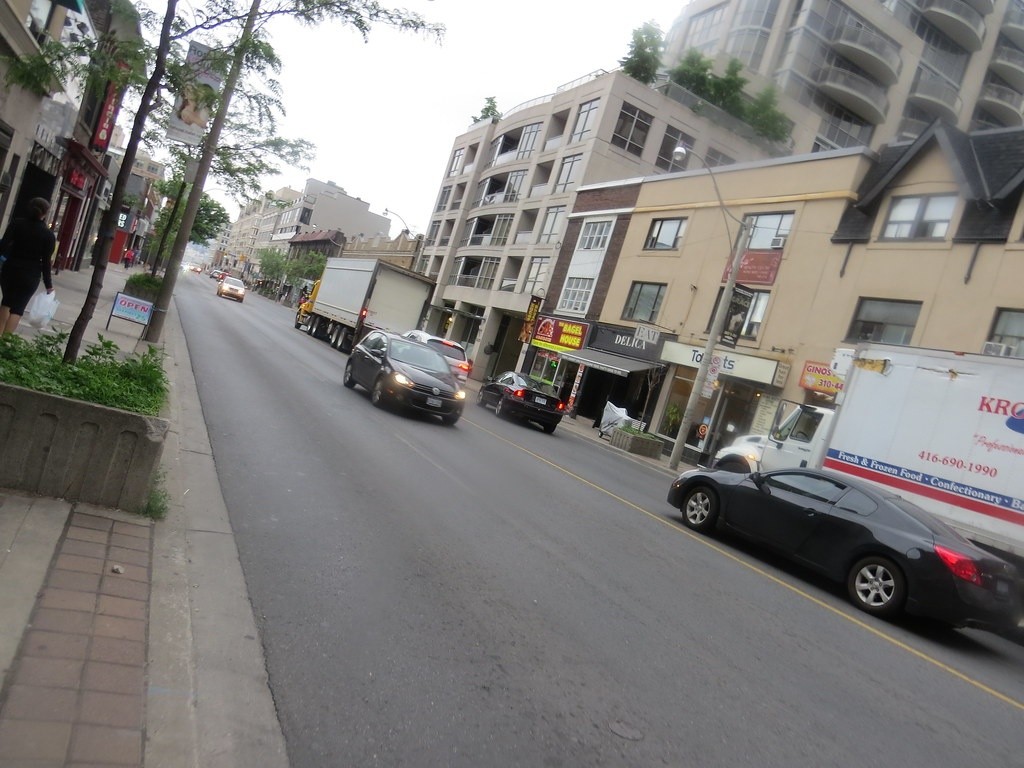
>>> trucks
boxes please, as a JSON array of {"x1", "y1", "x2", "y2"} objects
[{"x1": 710, "y1": 342, "x2": 1023, "y2": 573}]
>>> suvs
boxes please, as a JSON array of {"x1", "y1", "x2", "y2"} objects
[
  {"x1": 400, "y1": 329, "x2": 473, "y2": 388},
  {"x1": 344, "y1": 328, "x2": 467, "y2": 425}
]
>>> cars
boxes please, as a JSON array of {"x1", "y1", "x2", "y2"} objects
[
  {"x1": 217, "y1": 273, "x2": 231, "y2": 282},
  {"x1": 209, "y1": 270, "x2": 221, "y2": 279},
  {"x1": 184, "y1": 264, "x2": 202, "y2": 274},
  {"x1": 475, "y1": 370, "x2": 565, "y2": 434},
  {"x1": 215, "y1": 277, "x2": 248, "y2": 304},
  {"x1": 665, "y1": 465, "x2": 1024, "y2": 636}
]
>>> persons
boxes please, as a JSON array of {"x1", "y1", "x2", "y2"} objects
[
  {"x1": 124, "y1": 249, "x2": 140, "y2": 268},
  {"x1": 0, "y1": 197, "x2": 56, "y2": 339}
]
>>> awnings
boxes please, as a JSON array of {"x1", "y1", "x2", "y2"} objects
[{"x1": 558, "y1": 349, "x2": 661, "y2": 378}]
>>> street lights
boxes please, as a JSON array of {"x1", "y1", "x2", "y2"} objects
[
  {"x1": 270, "y1": 232, "x2": 294, "y2": 301},
  {"x1": 383, "y1": 206, "x2": 429, "y2": 272},
  {"x1": 311, "y1": 222, "x2": 344, "y2": 258},
  {"x1": 667, "y1": 145, "x2": 755, "y2": 469}
]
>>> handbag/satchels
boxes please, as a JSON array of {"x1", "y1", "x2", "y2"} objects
[{"x1": 28, "y1": 288, "x2": 60, "y2": 328}]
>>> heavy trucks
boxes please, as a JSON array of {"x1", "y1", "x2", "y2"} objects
[{"x1": 293, "y1": 256, "x2": 436, "y2": 353}]
